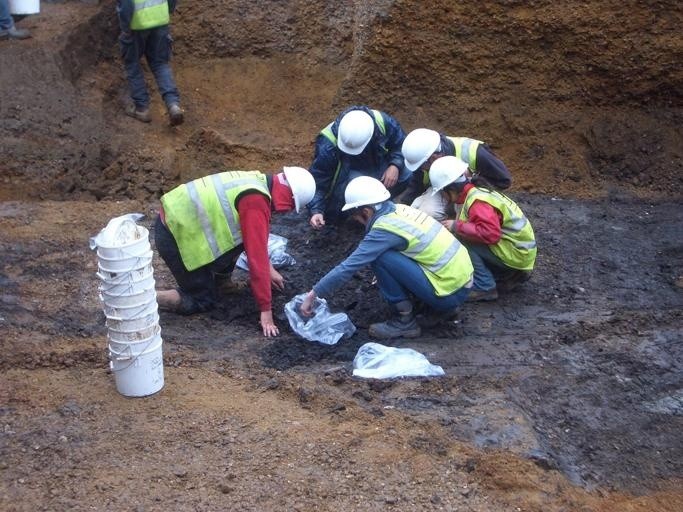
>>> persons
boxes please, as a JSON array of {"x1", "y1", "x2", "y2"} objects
[
  {"x1": 305, "y1": 105, "x2": 413, "y2": 231},
  {"x1": 0, "y1": 0, "x2": 32, "y2": 42},
  {"x1": 427, "y1": 155, "x2": 538, "y2": 303},
  {"x1": 115, "y1": 0, "x2": 185, "y2": 128},
  {"x1": 299, "y1": 175, "x2": 475, "y2": 339},
  {"x1": 154, "y1": 166, "x2": 316, "y2": 338},
  {"x1": 399, "y1": 127, "x2": 512, "y2": 223}
]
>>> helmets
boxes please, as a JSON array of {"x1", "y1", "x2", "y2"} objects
[
  {"x1": 283, "y1": 165, "x2": 317, "y2": 216},
  {"x1": 428, "y1": 155, "x2": 469, "y2": 197},
  {"x1": 336, "y1": 109, "x2": 374, "y2": 157},
  {"x1": 340, "y1": 175, "x2": 391, "y2": 211},
  {"x1": 401, "y1": 128, "x2": 441, "y2": 172}
]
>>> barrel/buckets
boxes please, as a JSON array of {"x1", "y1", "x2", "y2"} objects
[{"x1": 94, "y1": 225, "x2": 166, "y2": 398}]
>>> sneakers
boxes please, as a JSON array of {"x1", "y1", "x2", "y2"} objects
[
  {"x1": 168, "y1": 104, "x2": 185, "y2": 128},
  {"x1": 0, "y1": 26, "x2": 31, "y2": 40},
  {"x1": 467, "y1": 288, "x2": 499, "y2": 301},
  {"x1": 368, "y1": 317, "x2": 421, "y2": 340},
  {"x1": 125, "y1": 102, "x2": 151, "y2": 123}
]
